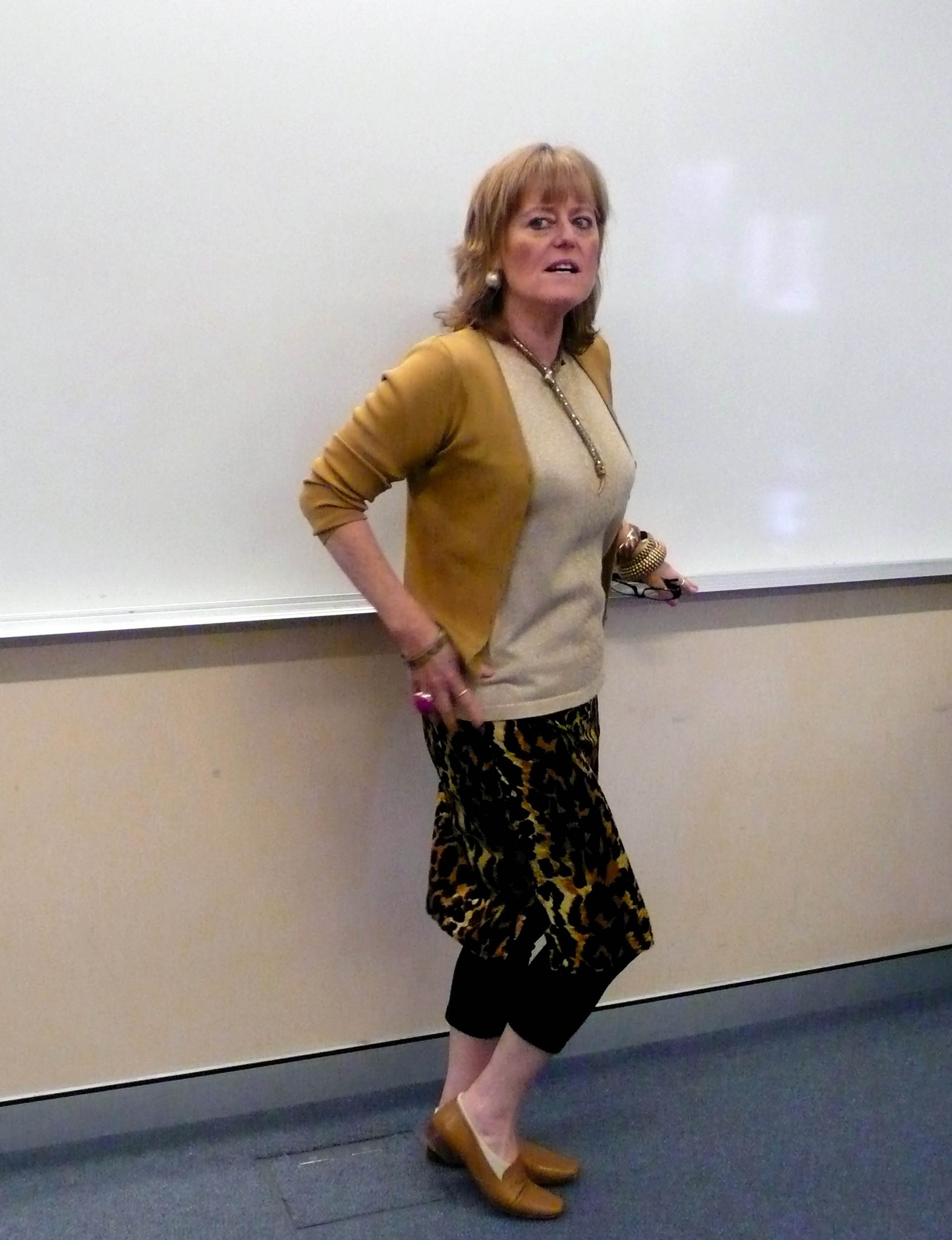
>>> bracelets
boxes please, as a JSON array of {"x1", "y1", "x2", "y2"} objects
[
  {"x1": 400, "y1": 629, "x2": 447, "y2": 666},
  {"x1": 616, "y1": 523, "x2": 667, "y2": 582}
]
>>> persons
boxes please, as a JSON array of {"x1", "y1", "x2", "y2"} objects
[{"x1": 299, "y1": 141, "x2": 699, "y2": 1219}]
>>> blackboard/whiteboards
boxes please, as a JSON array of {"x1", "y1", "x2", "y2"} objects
[{"x1": 2, "y1": 0, "x2": 951, "y2": 649}]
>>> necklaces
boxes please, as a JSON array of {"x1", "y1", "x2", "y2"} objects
[{"x1": 512, "y1": 334, "x2": 606, "y2": 495}]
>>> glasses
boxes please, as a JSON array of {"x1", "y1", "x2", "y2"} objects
[{"x1": 610, "y1": 578, "x2": 683, "y2": 600}]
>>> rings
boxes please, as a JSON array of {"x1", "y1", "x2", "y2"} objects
[
  {"x1": 412, "y1": 691, "x2": 434, "y2": 714},
  {"x1": 455, "y1": 689, "x2": 467, "y2": 699},
  {"x1": 680, "y1": 579, "x2": 685, "y2": 586}
]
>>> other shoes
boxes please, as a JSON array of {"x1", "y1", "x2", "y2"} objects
[
  {"x1": 425, "y1": 1116, "x2": 580, "y2": 1185},
  {"x1": 422, "y1": 1095, "x2": 565, "y2": 1218}
]
